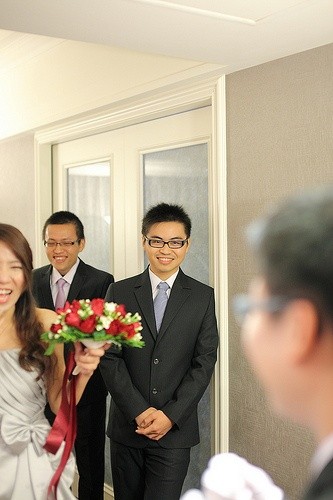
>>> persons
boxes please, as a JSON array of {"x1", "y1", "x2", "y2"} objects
[
  {"x1": 102, "y1": 201, "x2": 220, "y2": 500},
  {"x1": 31, "y1": 210, "x2": 115, "y2": 500},
  {"x1": 179, "y1": 181, "x2": 333, "y2": 500},
  {"x1": 0, "y1": 222, "x2": 113, "y2": 500}
]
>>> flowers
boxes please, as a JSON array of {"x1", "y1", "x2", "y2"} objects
[{"x1": 40, "y1": 299, "x2": 146, "y2": 375}]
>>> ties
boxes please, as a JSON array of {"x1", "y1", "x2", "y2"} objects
[
  {"x1": 55, "y1": 279, "x2": 68, "y2": 310},
  {"x1": 153, "y1": 282, "x2": 170, "y2": 332}
]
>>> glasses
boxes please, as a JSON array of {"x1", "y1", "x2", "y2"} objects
[
  {"x1": 144, "y1": 235, "x2": 190, "y2": 249},
  {"x1": 43, "y1": 239, "x2": 81, "y2": 247}
]
